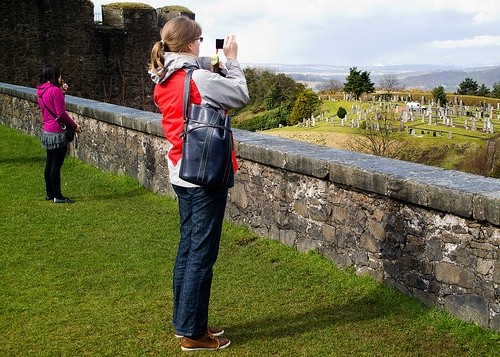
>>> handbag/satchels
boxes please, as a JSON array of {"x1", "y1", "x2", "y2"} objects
[
  {"x1": 55, "y1": 118, "x2": 77, "y2": 143},
  {"x1": 179, "y1": 104, "x2": 235, "y2": 188}
]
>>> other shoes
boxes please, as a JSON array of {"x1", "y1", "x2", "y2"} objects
[
  {"x1": 46, "y1": 197, "x2": 53, "y2": 200},
  {"x1": 180, "y1": 336, "x2": 231, "y2": 351},
  {"x1": 54, "y1": 197, "x2": 75, "y2": 203},
  {"x1": 174, "y1": 325, "x2": 224, "y2": 338}
]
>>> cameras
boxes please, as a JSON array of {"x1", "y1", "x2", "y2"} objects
[{"x1": 216, "y1": 39, "x2": 224, "y2": 49}]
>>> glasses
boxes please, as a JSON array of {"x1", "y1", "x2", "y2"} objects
[{"x1": 195, "y1": 36, "x2": 203, "y2": 42}]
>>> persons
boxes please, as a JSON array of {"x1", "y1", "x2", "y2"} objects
[
  {"x1": 146, "y1": 17, "x2": 249, "y2": 350},
  {"x1": 36, "y1": 63, "x2": 82, "y2": 203}
]
